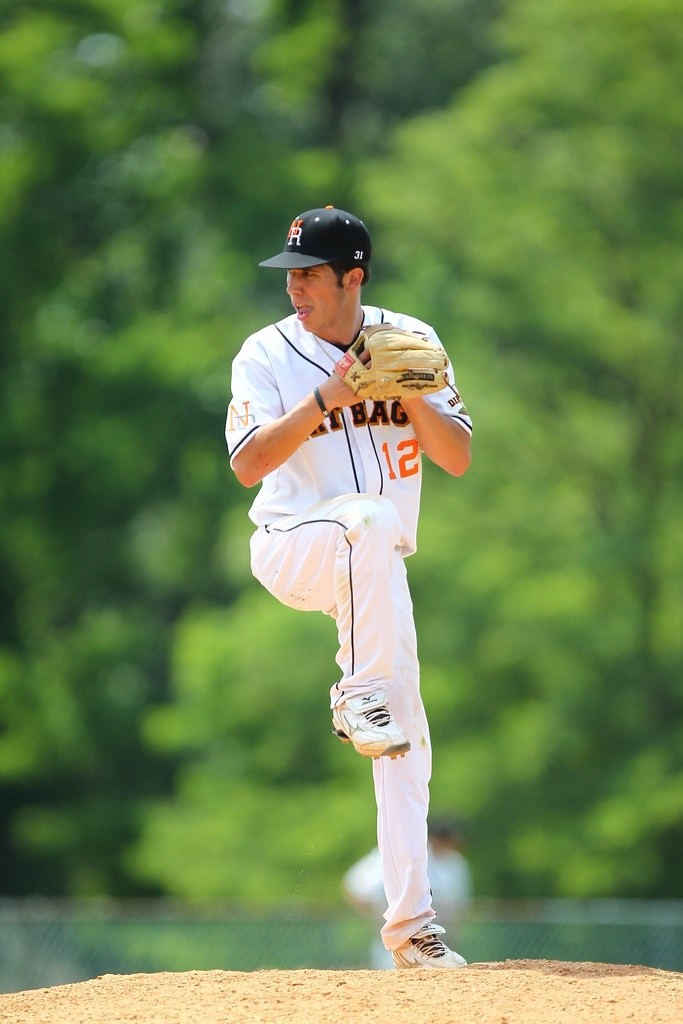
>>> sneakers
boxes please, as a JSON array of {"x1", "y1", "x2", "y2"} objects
[
  {"x1": 392, "y1": 924, "x2": 467, "y2": 971},
  {"x1": 331, "y1": 688, "x2": 411, "y2": 760}
]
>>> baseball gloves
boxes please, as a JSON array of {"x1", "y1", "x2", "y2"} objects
[{"x1": 334, "y1": 324, "x2": 449, "y2": 401}]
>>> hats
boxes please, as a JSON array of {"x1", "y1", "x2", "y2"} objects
[{"x1": 258, "y1": 206, "x2": 371, "y2": 268}]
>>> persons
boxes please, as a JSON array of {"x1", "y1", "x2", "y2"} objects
[
  {"x1": 224, "y1": 202, "x2": 476, "y2": 973},
  {"x1": 338, "y1": 811, "x2": 475, "y2": 971}
]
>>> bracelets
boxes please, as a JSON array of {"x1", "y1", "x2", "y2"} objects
[{"x1": 313, "y1": 386, "x2": 331, "y2": 418}]
